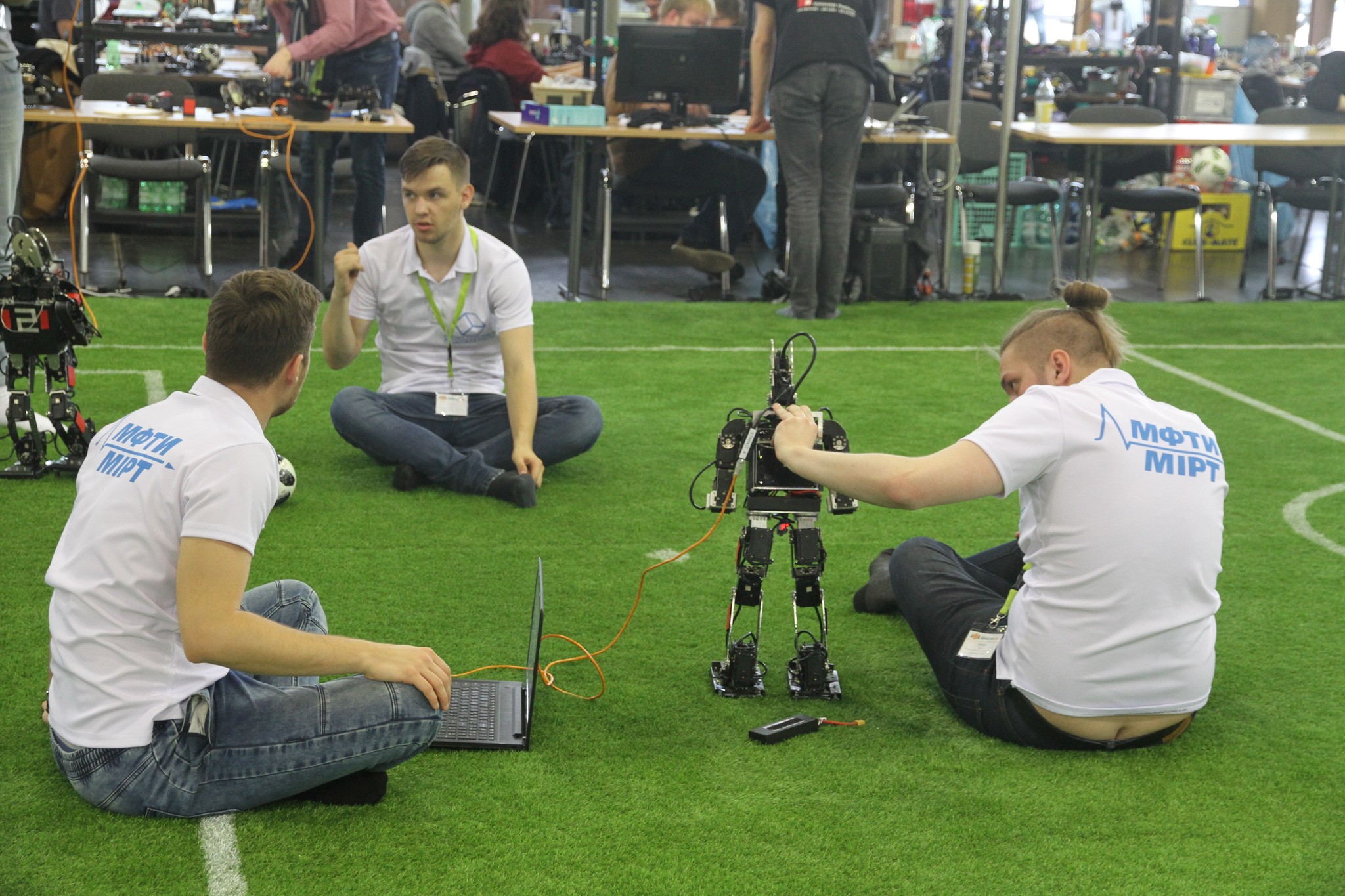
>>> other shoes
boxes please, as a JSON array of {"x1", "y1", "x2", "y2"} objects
[
  {"x1": 323, "y1": 271, "x2": 355, "y2": 298},
  {"x1": 471, "y1": 191, "x2": 493, "y2": 206},
  {"x1": 670, "y1": 242, "x2": 744, "y2": 280},
  {"x1": 278, "y1": 248, "x2": 314, "y2": 281}
]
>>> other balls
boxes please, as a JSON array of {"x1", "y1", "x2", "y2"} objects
[{"x1": 1189, "y1": 145, "x2": 1231, "y2": 186}]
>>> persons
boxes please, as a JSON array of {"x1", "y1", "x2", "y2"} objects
[
  {"x1": 324, "y1": 133, "x2": 605, "y2": 510},
  {"x1": 1, "y1": 0, "x2": 553, "y2": 302},
  {"x1": 1021, "y1": 0, "x2": 1047, "y2": 49},
  {"x1": 745, "y1": 0, "x2": 881, "y2": 321},
  {"x1": 1133, "y1": 3, "x2": 1194, "y2": 59},
  {"x1": 41, "y1": 265, "x2": 453, "y2": 820},
  {"x1": 770, "y1": 281, "x2": 1230, "y2": 754},
  {"x1": 603, "y1": 0, "x2": 768, "y2": 282}
]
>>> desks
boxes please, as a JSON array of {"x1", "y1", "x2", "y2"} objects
[{"x1": 0, "y1": 50, "x2": 1345, "y2": 306}]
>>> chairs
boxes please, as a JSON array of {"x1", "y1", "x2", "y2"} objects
[
  {"x1": 912, "y1": 99, "x2": 1063, "y2": 300},
  {"x1": 259, "y1": 128, "x2": 387, "y2": 270},
  {"x1": 1057, "y1": 104, "x2": 1205, "y2": 301},
  {"x1": 398, "y1": 46, "x2": 448, "y2": 149},
  {"x1": 1239, "y1": 70, "x2": 1285, "y2": 115},
  {"x1": 853, "y1": 102, "x2": 910, "y2": 300},
  {"x1": 79, "y1": 73, "x2": 216, "y2": 299},
  {"x1": 584, "y1": 23, "x2": 747, "y2": 307},
  {"x1": 448, "y1": 66, "x2": 517, "y2": 211},
  {"x1": 1238, "y1": 107, "x2": 1345, "y2": 301}
]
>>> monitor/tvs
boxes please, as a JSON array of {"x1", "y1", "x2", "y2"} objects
[{"x1": 614, "y1": 22, "x2": 743, "y2": 127}]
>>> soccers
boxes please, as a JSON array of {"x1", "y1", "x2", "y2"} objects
[{"x1": 273, "y1": 454, "x2": 297, "y2": 506}]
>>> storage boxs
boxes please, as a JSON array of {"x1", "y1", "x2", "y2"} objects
[{"x1": 1160, "y1": 184, "x2": 1253, "y2": 251}]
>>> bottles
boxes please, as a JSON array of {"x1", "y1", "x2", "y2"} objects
[
  {"x1": 964, "y1": 240, "x2": 981, "y2": 295},
  {"x1": 138, "y1": 181, "x2": 186, "y2": 214},
  {"x1": 1034, "y1": 73, "x2": 1055, "y2": 132},
  {"x1": 106, "y1": 39, "x2": 120, "y2": 69},
  {"x1": 1104, "y1": 1, "x2": 1126, "y2": 49},
  {"x1": 94, "y1": 175, "x2": 129, "y2": 209},
  {"x1": 134, "y1": 1, "x2": 143, "y2": 9}
]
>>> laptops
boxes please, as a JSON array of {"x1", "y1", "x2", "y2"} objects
[
  {"x1": 527, "y1": 20, "x2": 563, "y2": 52},
  {"x1": 430, "y1": 558, "x2": 543, "y2": 748}
]
